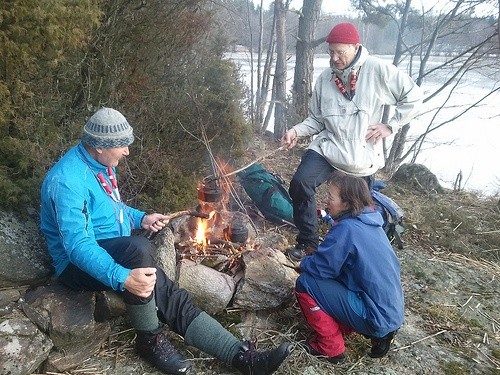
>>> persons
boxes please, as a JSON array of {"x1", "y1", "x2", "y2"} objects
[
  {"x1": 280, "y1": 22, "x2": 423, "y2": 262},
  {"x1": 295, "y1": 171, "x2": 404, "y2": 364},
  {"x1": 40, "y1": 107, "x2": 292, "y2": 375}
]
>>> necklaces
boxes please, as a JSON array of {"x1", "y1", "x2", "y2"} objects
[
  {"x1": 95, "y1": 164, "x2": 122, "y2": 203},
  {"x1": 332, "y1": 70, "x2": 357, "y2": 101}
]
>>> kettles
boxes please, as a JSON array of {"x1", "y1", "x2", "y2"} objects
[
  {"x1": 223, "y1": 216, "x2": 248, "y2": 243},
  {"x1": 197, "y1": 178, "x2": 223, "y2": 202}
]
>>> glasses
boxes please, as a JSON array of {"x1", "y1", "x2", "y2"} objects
[{"x1": 327, "y1": 47, "x2": 352, "y2": 57}]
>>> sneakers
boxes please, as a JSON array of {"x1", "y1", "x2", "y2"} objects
[
  {"x1": 234, "y1": 341, "x2": 292, "y2": 375},
  {"x1": 135, "y1": 330, "x2": 192, "y2": 375}
]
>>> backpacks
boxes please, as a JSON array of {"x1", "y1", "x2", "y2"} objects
[
  {"x1": 371, "y1": 188, "x2": 406, "y2": 249},
  {"x1": 238, "y1": 161, "x2": 293, "y2": 224}
]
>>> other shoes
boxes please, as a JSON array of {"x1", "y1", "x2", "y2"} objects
[
  {"x1": 305, "y1": 338, "x2": 348, "y2": 365},
  {"x1": 369, "y1": 328, "x2": 396, "y2": 357},
  {"x1": 286, "y1": 244, "x2": 312, "y2": 260}
]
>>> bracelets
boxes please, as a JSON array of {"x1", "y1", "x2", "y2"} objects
[{"x1": 386, "y1": 124, "x2": 392, "y2": 131}]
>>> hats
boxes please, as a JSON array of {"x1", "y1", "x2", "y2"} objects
[
  {"x1": 327, "y1": 23, "x2": 360, "y2": 44},
  {"x1": 82, "y1": 108, "x2": 134, "y2": 149}
]
